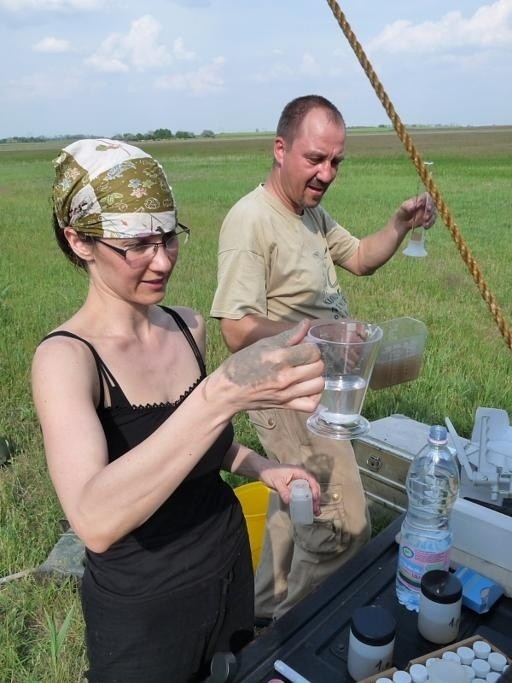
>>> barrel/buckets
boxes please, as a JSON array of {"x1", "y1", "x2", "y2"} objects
[{"x1": 232, "y1": 480, "x2": 273, "y2": 569}]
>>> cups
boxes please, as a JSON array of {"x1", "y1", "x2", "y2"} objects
[{"x1": 305, "y1": 320, "x2": 385, "y2": 441}]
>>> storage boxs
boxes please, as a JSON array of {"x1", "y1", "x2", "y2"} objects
[
  {"x1": 361, "y1": 633, "x2": 512, "y2": 683},
  {"x1": 352, "y1": 413, "x2": 471, "y2": 520}
]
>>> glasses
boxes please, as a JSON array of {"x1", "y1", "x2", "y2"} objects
[{"x1": 93, "y1": 221, "x2": 192, "y2": 267}]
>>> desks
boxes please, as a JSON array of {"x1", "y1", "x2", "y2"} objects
[{"x1": 205, "y1": 510, "x2": 512, "y2": 682}]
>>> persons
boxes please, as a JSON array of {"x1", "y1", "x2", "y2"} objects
[
  {"x1": 32, "y1": 135, "x2": 327, "y2": 683},
  {"x1": 208, "y1": 94, "x2": 438, "y2": 634}
]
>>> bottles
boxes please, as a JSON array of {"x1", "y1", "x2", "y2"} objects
[
  {"x1": 401, "y1": 160, "x2": 437, "y2": 258},
  {"x1": 393, "y1": 424, "x2": 462, "y2": 616},
  {"x1": 288, "y1": 477, "x2": 315, "y2": 531},
  {"x1": 343, "y1": 607, "x2": 399, "y2": 682},
  {"x1": 413, "y1": 569, "x2": 465, "y2": 644}
]
